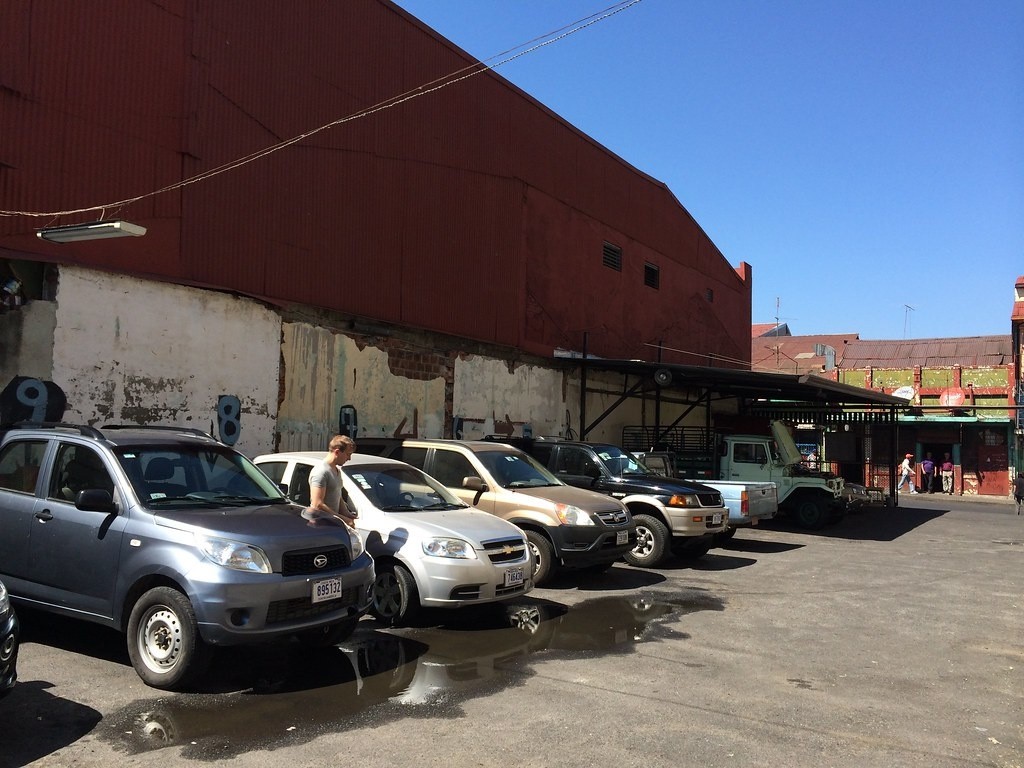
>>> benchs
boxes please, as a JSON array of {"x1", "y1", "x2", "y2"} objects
[{"x1": 1, "y1": 465, "x2": 143, "y2": 496}]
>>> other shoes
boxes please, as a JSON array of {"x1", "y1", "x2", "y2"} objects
[
  {"x1": 949, "y1": 492, "x2": 952, "y2": 495},
  {"x1": 928, "y1": 490, "x2": 931, "y2": 494},
  {"x1": 910, "y1": 491, "x2": 918, "y2": 495},
  {"x1": 942, "y1": 490, "x2": 947, "y2": 493},
  {"x1": 895, "y1": 489, "x2": 901, "y2": 494}
]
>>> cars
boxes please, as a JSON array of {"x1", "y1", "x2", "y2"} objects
[
  {"x1": 841, "y1": 483, "x2": 867, "y2": 507},
  {"x1": 251, "y1": 449, "x2": 537, "y2": 621},
  {"x1": 1, "y1": 576, "x2": 22, "y2": 696}
]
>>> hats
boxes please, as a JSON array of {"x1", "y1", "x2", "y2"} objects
[
  {"x1": 812, "y1": 449, "x2": 816, "y2": 452},
  {"x1": 905, "y1": 454, "x2": 913, "y2": 458}
]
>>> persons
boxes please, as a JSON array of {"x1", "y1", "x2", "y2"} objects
[
  {"x1": 807, "y1": 449, "x2": 824, "y2": 471},
  {"x1": 308, "y1": 435, "x2": 355, "y2": 530},
  {"x1": 895, "y1": 454, "x2": 918, "y2": 495},
  {"x1": 939, "y1": 451, "x2": 954, "y2": 496},
  {"x1": 1013, "y1": 471, "x2": 1024, "y2": 515},
  {"x1": 921, "y1": 451, "x2": 937, "y2": 494}
]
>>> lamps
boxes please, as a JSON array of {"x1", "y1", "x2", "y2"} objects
[{"x1": 37, "y1": 219, "x2": 148, "y2": 244}]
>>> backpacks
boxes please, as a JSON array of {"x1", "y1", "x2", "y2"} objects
[{"x1": 898, "y1": 464, "x2": 902, "y2": 475}]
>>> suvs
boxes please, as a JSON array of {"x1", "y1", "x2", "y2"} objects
[
  {"x1": 529, "y1": 439, "x2": 739, "y2": 572},
  {"x1": 348, "y1": 436, "x2": 638, "y2": 589},
  {"x1": 1, "y1": 419, "x2": 380, "y2": 688}
]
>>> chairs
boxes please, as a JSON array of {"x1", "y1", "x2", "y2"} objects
[
  {"x1": 144, "y1": 457, "x2": 189, "y2": 500},
  {"x1": 61, "y1": 455, "x2": 95, "y2": 500}
]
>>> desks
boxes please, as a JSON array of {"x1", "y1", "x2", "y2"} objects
[{"x1": 867, "y1": 487, "x2": 883, "y2": 507}]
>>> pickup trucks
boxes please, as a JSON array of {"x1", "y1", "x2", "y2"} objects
[
  {"x1": 638, "y1": 452, "x2": 776, "y2": 541},
  {"x1": 621, "y1": 425, "x2": 844, "y2": 531}
]
